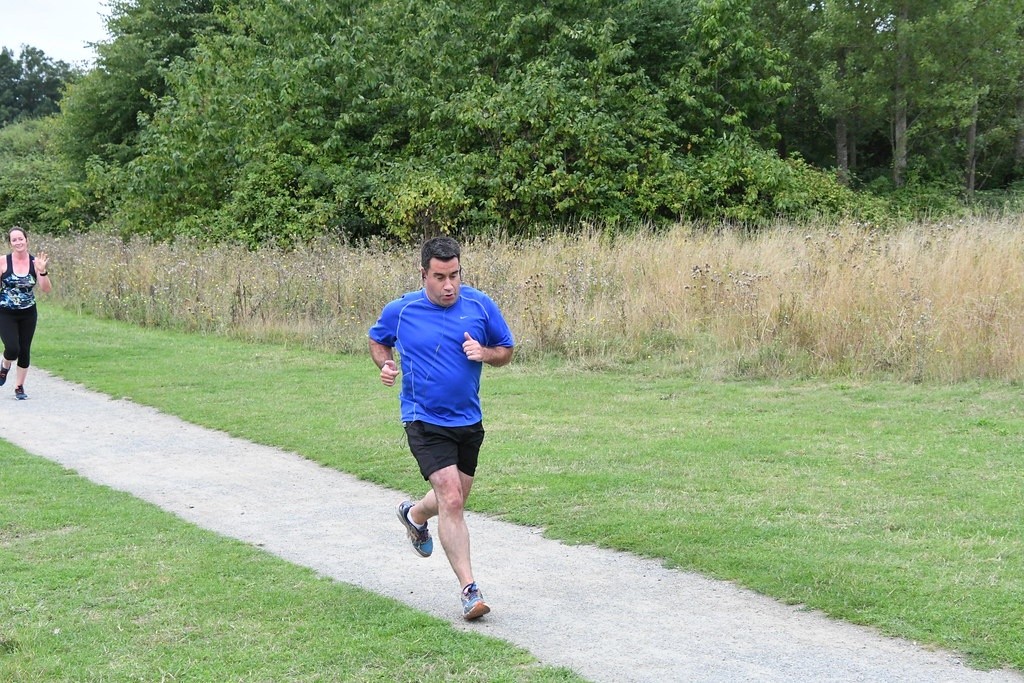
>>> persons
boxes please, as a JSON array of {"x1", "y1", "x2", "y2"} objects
[
  {"x1": 369, "y1": 237, "x2": 514, "y2": 619},
  {"x1": 0, "y1": 226, "x2": 52, "y2": 401}
]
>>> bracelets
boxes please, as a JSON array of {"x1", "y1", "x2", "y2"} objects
[{"x1": 40, "y1": 270, "x2": 48, "y2": 276}]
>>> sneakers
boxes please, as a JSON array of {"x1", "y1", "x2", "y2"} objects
[
  {"x1": 15, "y1": 386, "x2": 27, "y2": 400},
  {"x1": 460, "y1": 581, "x2": 490, "y2": 618},
  {"x1": 395, "y1": 500, "x2": 434, "y2": 557},
  {"x1": 0, "y1": 359, "x2": 12, "y2": 386}
]
